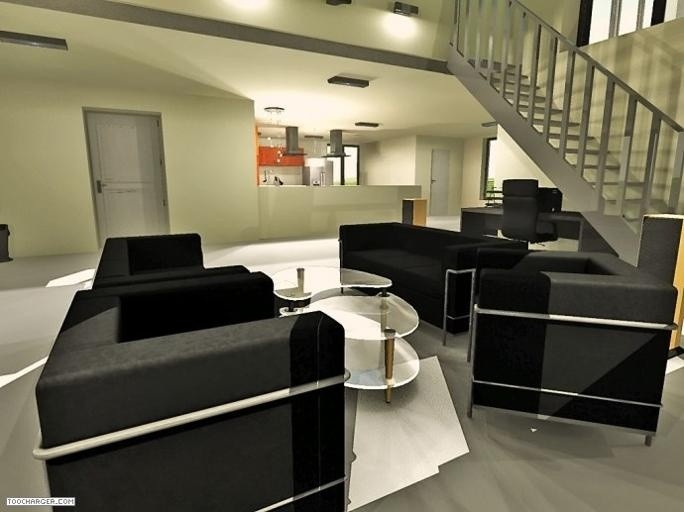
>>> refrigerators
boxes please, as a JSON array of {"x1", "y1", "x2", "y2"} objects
[{"x1": 309, "y1": 159, "x2": 333, "y2": 185}]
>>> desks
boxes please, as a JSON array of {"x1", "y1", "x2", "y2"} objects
[{"x1": 460, "y1": 207, "x2": 618, "y2": 258}]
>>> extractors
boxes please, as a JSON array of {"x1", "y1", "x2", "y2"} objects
[
  {"x1": 283, "y1": 127, "x2": 308, "y2": 156},
  {"x1": 321, "y1": 129, "x2": 351, "y2": 157}
]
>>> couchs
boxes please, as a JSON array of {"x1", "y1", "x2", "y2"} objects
[{"x1": 338, "y1": 223, "x2": 529, "y2": 346}]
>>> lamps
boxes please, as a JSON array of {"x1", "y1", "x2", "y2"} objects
[{"x1": 262, "y1": 106, "x2": 285, "y2": 120}]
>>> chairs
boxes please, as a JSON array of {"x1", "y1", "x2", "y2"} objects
[
  {"x1": 467, "y1": 245, "x2": 680, "y2": 447},
  {"x1": 30, "y1": 271, "x2": 352, "y2": 512},
  {"x1": 93, "y1": 232, "x2": 252, "y2": 288},
  {"x1": 502, "y1": 179, "x2": 563, "y2": 245}
]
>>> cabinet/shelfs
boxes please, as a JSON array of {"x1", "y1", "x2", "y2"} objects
[{"x1": 402, "y1": 200, "x2": 427, "y2": 226}]
